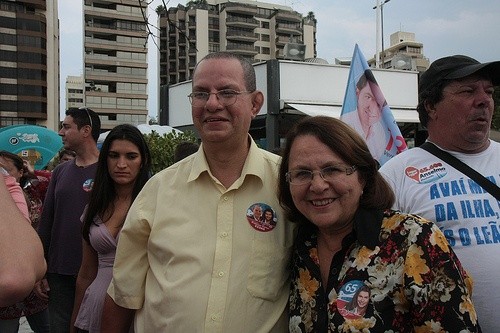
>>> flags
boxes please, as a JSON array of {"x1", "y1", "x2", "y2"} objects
[{"x1": 339, "y1": 44, "x2": 410, "y2": 168}]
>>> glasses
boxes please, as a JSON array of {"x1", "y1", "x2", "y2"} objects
[
  {"x1": 285, "y1": 165, "x2": 359, "y2": 185},
  {"x1": 78, "y1": 106, "x2": 92, "y2": 127},
  {"x1": 187, "y1": 88, "x2": 252, "y2": 107}
]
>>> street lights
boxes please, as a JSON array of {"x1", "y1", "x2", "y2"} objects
[{"x1": 372, "y1": 0, "x2": 391, "y2": 69}]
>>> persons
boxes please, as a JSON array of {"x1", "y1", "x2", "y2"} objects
[
  {"x1": 247, "y1": 205, "x2": 263, "y2": 222},
  {"x1": 0, "y1": 149, "x2": 55, "y2": 333},
  {"x1": 259, "y1": 208, "x2": 277, "y2": 226},
  {"x1": 98, "y1": 50, "x2": 298, "y2": 333},
  {"x1": 277, "y1": 115, "x2": 484, "y2": 333},
  {"x1": 338, "y1": 69, "x2": 388, "y2": 162},
  {"x1": 70, "y1": 123, "x2": 152, "y2": 333},
  {"x1": 60, "y1": 149, "x2": 76, "y2": 164},
  {"x1": 376, "y1": 55, "x2": 500, "y2": 333},
  {"x1": 344, "y1": 285, "x2": 374, "y2": 319},
  {"x1": 36, "y1": 103, "x2": 101, "y2": 333}
]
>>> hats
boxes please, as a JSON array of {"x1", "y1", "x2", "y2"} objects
[{"x1": 418, "y1": 55, "x2": 500, "y2": 94}]
